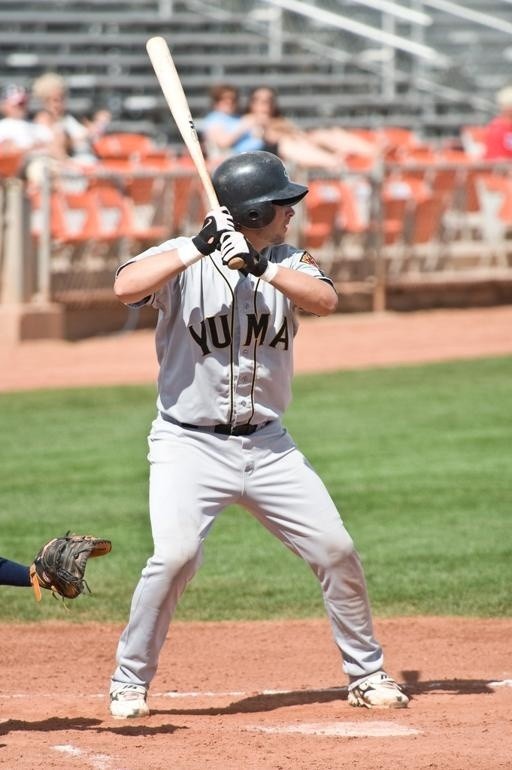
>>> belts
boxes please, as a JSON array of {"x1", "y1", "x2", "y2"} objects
[{"x1": 182, "y1": 421, "x2": 272, "y2": 436}]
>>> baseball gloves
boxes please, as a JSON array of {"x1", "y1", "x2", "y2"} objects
[{"x1": 30, "y1": 529, "x2": 112, "y2": 601}]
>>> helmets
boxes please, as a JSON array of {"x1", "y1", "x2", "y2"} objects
[{"x1": 211, "y1": 151, "x2": 309, "y2": 229}]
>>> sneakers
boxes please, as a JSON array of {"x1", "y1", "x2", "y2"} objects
[
  {"x1": 348, "y1": 670, "x2": 409, "y2": 708},
  {"x1": 108, "y1": 685, "x2": 150, "y2": 719}
]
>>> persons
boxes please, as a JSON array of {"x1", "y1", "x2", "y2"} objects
[
  {"x1": 473, "y1": 86, "x2": 511, "y2": 178},
  {"x1": 0, "y1": 556, "x2": 50, "y2": 587},
  {"x1": 1, "y1": 75, "x2": 155, "y2": 237},
  {"x1": 107, "y1": 152, "x2": 410, "y2": 717},
  {"x1": 200, "y1": 85, "x2": 346, "y2": 172}
]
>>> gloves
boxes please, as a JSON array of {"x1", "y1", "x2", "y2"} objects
[{"x1": 178, "y1": 207, "x2": 278, "y2": 283}]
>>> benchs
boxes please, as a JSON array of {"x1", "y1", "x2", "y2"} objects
[{"x1": 0, "y1": 1, "x2": 512, "y2": 255}]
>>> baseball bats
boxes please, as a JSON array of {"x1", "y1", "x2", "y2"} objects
[{"x1": 145, "y1": 35, "x2": 245, "y2": 270}]
